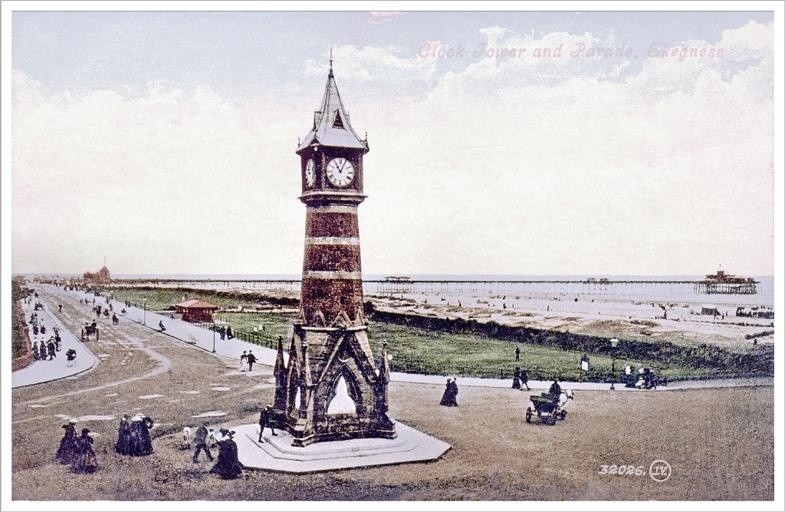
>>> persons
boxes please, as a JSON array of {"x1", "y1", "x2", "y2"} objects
[
  {"x1": 28, "y1": 298, "x2": 132, "y2": 368},
  {"x1": 247, "y1": 350, "x2": 258, "y2": 371},
  {"x1": 241, "y1": 350, "x2": 248, "y2": 371},
  {"x1": 448, "y1": 381, "x2": 459, "y2": 407},
  {"x1": 521, "y1": 370, "x2": 530, "y2": 391},
  {"x1": 258, "y1": 405, "x2": 278, "y2": 442},
  {"x1": 220, "y1": 326, "x2": 226, "y2": 340},
  {"x1": 516, "y1": 346, "x2": 520, "y2": 361},
  {"x1": 512, "y1": 367, "x2": 522, "y2": 389},
  {"x1": 440, "y1": 379, "x2": 453, "y2": 406},
  {"x1": 226, "y1": 326, "x2": 233, "y2": 339},
  {"x1": 55, "y1": 413, "x2": 245, "y2": 479},
  {"x1": 548, "y1": 379, "x2": 561, "y2": 397}
]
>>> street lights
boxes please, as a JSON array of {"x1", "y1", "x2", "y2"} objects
[
  {"x1": 142, "y1": 297, "x2": 147, "y2": 325},
  {"x1": 610, "y1": 336, "x2": 619, "y2": 390},
  {"x1": 376, "y1": 337, "x2": 384, "y2": 360},
  {"x1": 210, "y1": 312, "x2": 217, "y2": 353}
]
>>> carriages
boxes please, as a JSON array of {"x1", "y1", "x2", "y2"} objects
[
  {"x1": 80, "y1": 321, "x2": 99, "y2": 342},
  {"x1": 525, "y1": 388, "x2": 575, "y2": 425}
]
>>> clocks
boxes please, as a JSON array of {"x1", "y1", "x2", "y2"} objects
[
  {"x1": 326, "y1": 157, "x2": 355, "y2": 189},
  {"x1": 303, "y1": 159, "x2": 318, "y2": 187}
]
